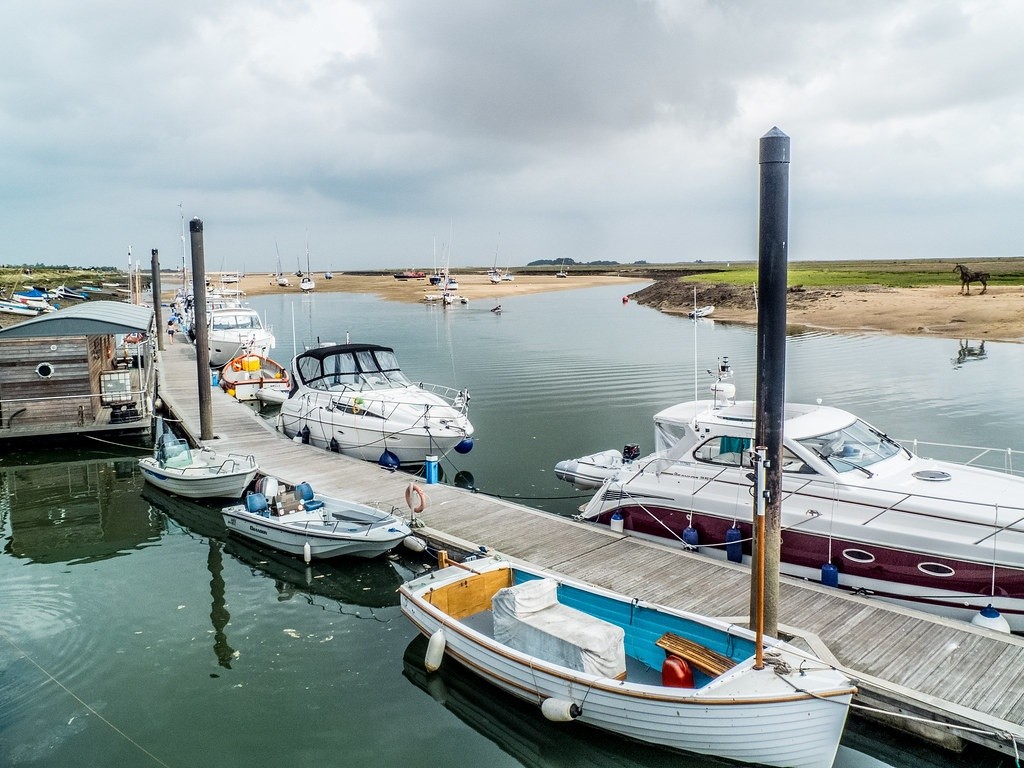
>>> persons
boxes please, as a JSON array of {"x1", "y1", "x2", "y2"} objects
[{"x1": 166, "y1": 320, "x2": 175, "y2": 345}]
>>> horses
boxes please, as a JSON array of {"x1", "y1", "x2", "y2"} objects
[{"x1": 953, "y1": 262, "x2": 990, "y2": 295}]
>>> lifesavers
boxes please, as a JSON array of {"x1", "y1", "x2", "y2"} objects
[
  {"x1": 232, "y1": 358, "x2": 242, "y2": 371},
  {"x1": 405, "y1": 484, "x2": 425, "y2": 513}
]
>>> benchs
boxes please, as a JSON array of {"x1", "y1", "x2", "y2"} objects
[{"x1": 656, "y1": 633, "x2": 738, "y2": 676}]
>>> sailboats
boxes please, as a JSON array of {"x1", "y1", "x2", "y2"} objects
[
  {"x1": 300, "y1": 241, "x2": 315, "y2": 290},
  {"x1": 296, "y1": 256, "x2": 304, "y2": 277},
  {"x1": 556, "y1": 255, "x2": 568, "y2": 278},
  {"x1": 392, "y1": 227, "x2": 514, "y2": 306},
  {"x1": 275, "y1": 242, "x2": 289, "y2": 286}
]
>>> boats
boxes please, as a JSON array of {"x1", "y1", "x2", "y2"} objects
[
  {"x1": 136, "y1": 432, "x2": 260, "y2": 500},
  {"x1": 552, "y1": 287, "x2": 1024, "y2": 638},
  {"x1": 325, "y1": 272, "x2": 333, "y2": 280},
  {"x1": 169, "y1": 216, "x2": 276, "y2": 365},
  {"x1": 271, "y1": 300, "x2": 477, "y2": 472},
  {"x1": 219, "y1": 474, "x2": 415, "y2": 562},
  {"x1": 394, "y1": 445, "x2": 861, "y2": 768},
  {"x1": 219, "y1": 336, "x2": 291, "y2": 406},
  {"x1": 0, "y1": 265, "x2": 132, "y2": 317},
  {"x1": 401, "y1": 629, "x2": 777, "y2": 768},
  {"x1": 222, "y1": 530, "x2": 402, "y2": 610},
  {"x1": 139, "y1": 479, "x2": 245, "y2": 543},
  {"x1": 688, "y1": 305, "x2": 715, "y2": 319}
]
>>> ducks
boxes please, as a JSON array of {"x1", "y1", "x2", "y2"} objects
[{"x1": 490, "y1": 304, "x2": 502, "y2": 313}]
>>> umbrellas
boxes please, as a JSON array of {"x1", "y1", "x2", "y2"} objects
[{"x1": 168, "y1": 316, "x2": 177, "y2": 323}]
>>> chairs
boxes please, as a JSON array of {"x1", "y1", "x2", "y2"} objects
[
  {"x1": 295, "y1": 483, "x2": 323, "y2": 511},
  {"x1": 247, "y1": 493, "x2": 270, "y2": 519}
]
